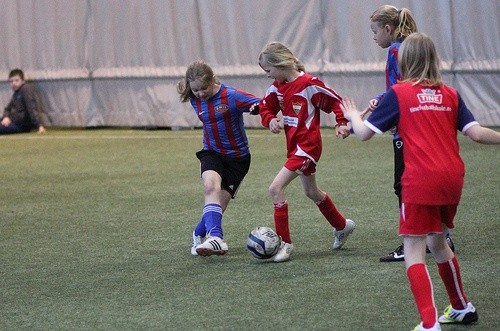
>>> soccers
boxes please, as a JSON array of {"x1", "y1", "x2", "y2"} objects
[{"x1": 246, "y1": 227, "x2": 280, "y2": 260}]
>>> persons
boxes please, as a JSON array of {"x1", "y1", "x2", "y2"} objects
[
  {"x1": 0, "y1": 69, "x2": 45, "y2": 134},
  {"x1": 362, "y1": 5, "x2": 454, "y2": 262},
  {"x1": 258, "y1": 42, "x2": 356, "y2": 261},
  {"x1": 176, "y1": 62, "x2": 261, "y2": 256},
  {"x1": 340, "y1": 33, "x2": 500, "y2": 331}
]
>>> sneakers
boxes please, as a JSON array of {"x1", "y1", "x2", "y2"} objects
[
  {"x1": 191, "y1": 230, "x2": 205, "y2": 255},
  {"x1": 196, "y1": 233, "x2": 228, "y2": 257},
  {"x1": 438, "y1": 302, "x2": 479, "y2": 323},
  {"x1": 413, "y1": 321, "x2": 441, "y2": 331},
  {"x1": 379, "y1": 244, "x2": 406, "y2": 262},
  {"x1": 332, "y1": 219, "x2": 355, "y2": 250},
  {"x1": 273, "y1": 236, "x2": 294, "y2": 263},
  {"x1": 425, "y1": 238, "x2": 455, "y2": 253}
]
